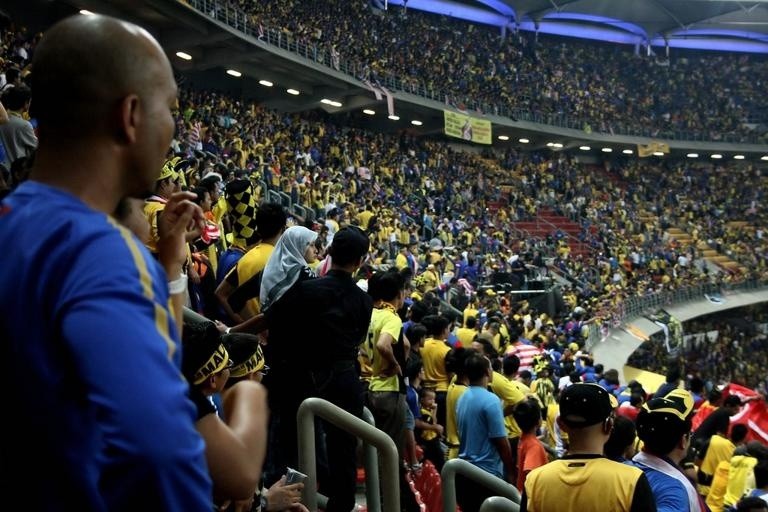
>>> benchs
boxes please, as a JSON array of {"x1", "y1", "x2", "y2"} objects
[{"x1": 488, "y1": 183, "x2": 766, "y2": 275}]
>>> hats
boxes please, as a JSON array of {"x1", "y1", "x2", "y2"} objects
[
  {"x1": 559, "y1": 382, "x2": 618, "y2": 428},
  {"x1": 259, "y1": 225, "x2": 319, "y2": 312},
  {"x1": 636, "y1": 388, "x2": 695, "y2": 444}
]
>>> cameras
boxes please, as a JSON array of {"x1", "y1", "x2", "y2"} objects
[{"x1": 284, "y1": 465, "x2": 310, "y2": 492}]
